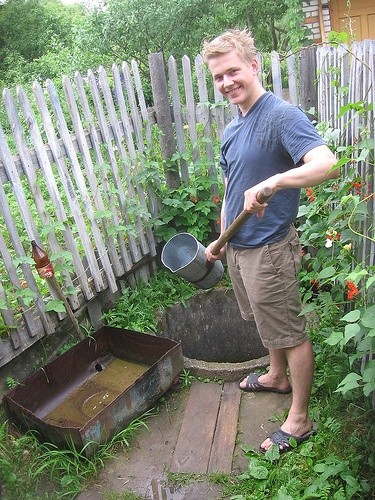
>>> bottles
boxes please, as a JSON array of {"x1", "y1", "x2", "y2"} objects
[{"x1": 31, "y1": 240, "x2": 54, "y2": 279}]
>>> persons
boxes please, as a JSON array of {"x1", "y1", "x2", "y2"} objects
[{"x1": 200, "y1": 25, "x2": 342, "y2": 457}]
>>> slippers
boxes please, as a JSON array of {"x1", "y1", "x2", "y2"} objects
[
  {"x1": 238, "y1": 371, "x2": 291, "y2": 396},
  {"x1": 258, "y1": 419, "x2": 315, "y2": 457}
]
